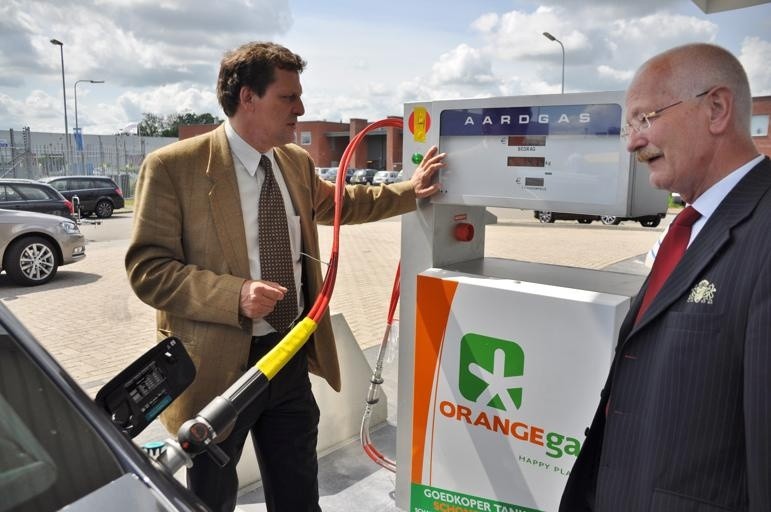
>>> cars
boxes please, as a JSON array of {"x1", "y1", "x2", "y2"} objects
[
  {"x1": 0, "y1": 179, "x2": 74, "y2": 219},
  {"x1": 315, "y1": 166, "x2": 399, "y2": 185},
  {"x1": 0, "y1": 209, "x2": 86, "y2": 286}
]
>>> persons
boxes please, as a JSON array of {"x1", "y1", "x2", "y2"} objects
[
  {"x1": 125, "y1": 41, "x2": 447, "y2": 512},
  {"x1": 557, "y1": 42, "x2": 771, "y2": 512}
]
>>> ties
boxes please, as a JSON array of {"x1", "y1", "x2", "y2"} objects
[
  {"x1": 606, "y1": 207, "x2": 704, "y2": 418},
  {"x1": 259, "y1": 156, "x2": 298, "y2": 335}
]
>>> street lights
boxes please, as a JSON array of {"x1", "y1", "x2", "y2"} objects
[
  {"x1": 543, "y1": 32, "x2": 565, "y2": 94},
  {"x1": 49, "y1": 38, "x2": 69, "y2": 150},
  {"x1": 75, "y1": 79, "x2": 104, "y2": 134}
]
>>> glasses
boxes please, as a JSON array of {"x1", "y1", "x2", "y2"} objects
[{"x1": 620, "y1": 87, "x2": 713, "y2": 137}]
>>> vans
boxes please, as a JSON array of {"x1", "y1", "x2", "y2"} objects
[{"x1": 38, "y1": 175, "x2": 124, "y2": 219}]
